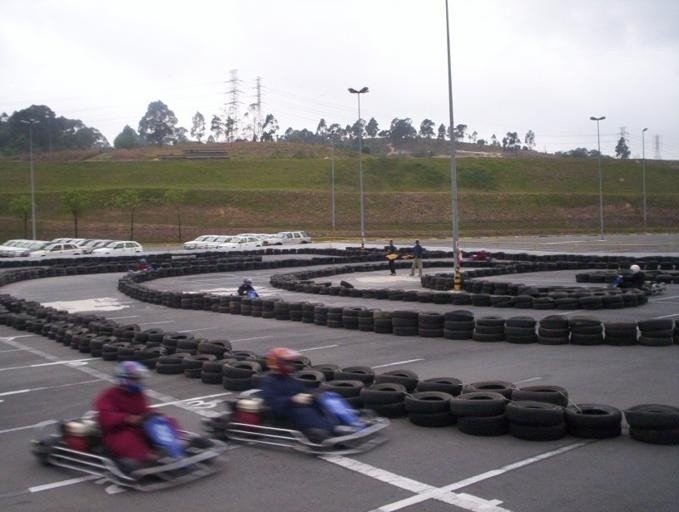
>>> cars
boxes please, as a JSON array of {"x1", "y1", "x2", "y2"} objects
[
  {"x1": 0, "y1": 236, "x2": 146, "y2": 261},
  {"x1": 182, "y1": 230, "x2": 311, "y2": 252}
]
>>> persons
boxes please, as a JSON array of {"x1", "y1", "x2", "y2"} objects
[
  {"x1": 607, "y1": 264, "x2": 644, "y2": 289},
  {"x1": 387, "y1": 240, "x2": 397, "y2": 275},
  {"x1": 261, "y1": 348, "x2": 372, "y2": 434},
  {"x1": 139, "y1": 259, "x2": 149, "y2": 271},
  {"x1": 91, "y1": 360, "x2": 189, "y2": 463},
  {"x1": 409, "y1": 240, "x2": 423, "y2": 277},
  {"x1": 238, "y1": 278, "x2": 259, "y2": 297}
]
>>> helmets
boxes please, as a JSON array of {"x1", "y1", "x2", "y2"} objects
[
  {"x1": 139, "y1": 258, "x2": 146, "y2": 264},
  {"x1": 243, "y1": 277, "x2": 253, "y2": 286},
  {"x1": 113, "y1": 360, "x2": 151, "y2": 391},
  {"x1": 267, "y1": 347, "x2": 309, "y2": 375},
  {"x1": 630, "y1": 263, "x2": 641, "y2": 274}
]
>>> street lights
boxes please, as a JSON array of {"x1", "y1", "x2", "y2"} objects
[
  {"x1": 589, "y1": 115, "x2": 607, "y2": 242},
  {"x1": 20, "y1": 118, "x2": 40, "y2": 242},
  {"x1": 324, "y1": 133, "x2": 341, "y2": 230},
  {"x1": 348, "y1": 85, "x2": 369, "y2": 248},
  {"x1": 641, "y1": 127, "x2": 648, "y2": 236}
]
